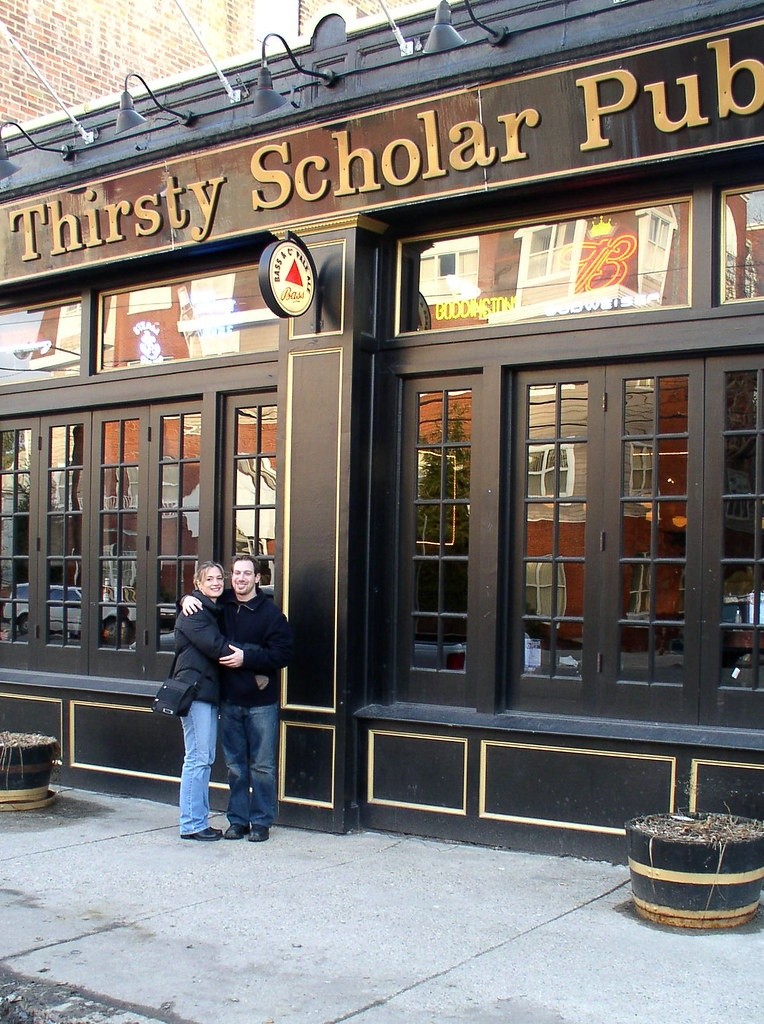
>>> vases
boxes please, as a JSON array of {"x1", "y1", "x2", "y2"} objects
[
  {"x1": 0, "y1": 731, "x2": 61, "y2": 812},
  {"x1": 624, "y1": 811, "x2": 764, "y2": 931}
]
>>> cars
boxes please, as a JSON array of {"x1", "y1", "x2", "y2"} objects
[
  {"x1": 128, "y1": 584, "x2": 274, "y2": 653},
  {"x1": 414, "y1": 560, "x2": 543, "y2": 673},
  {"x1": 4, "y1": 582, "x2": 136, "y2": 644}
]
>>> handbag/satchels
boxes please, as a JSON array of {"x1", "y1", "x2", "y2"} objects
[{"x1": 152, "y1": 679, "x2": 196, "y2": 718}]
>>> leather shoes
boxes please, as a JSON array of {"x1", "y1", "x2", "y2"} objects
[
  {"x1": 249, "y1": 823, "x2": 269, "y2": 842},
  {"x1": 207, "y1": 827, "x2": 223, "y2": 837},
  {"x1": 180, "y1": 828, "x2": 221, "y2": 841},
  {"x1": 224, "y1": 823, "x2": 250, "y2": 840}
]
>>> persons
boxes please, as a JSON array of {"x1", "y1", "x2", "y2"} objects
[{"x1": 174, "y1": 554, "x2": 292, "y2": 842}]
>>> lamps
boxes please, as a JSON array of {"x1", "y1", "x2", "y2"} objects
[
  {"x1": 115, "y1": 73, "x2": 192, "y2": 135},
  {"x1": 250, "y1": 33, "x2": 335, "y2": 118},
  {"x1": 0, "y1": 122, "x2": 72, "y2": 160},
  {"x1": 421, "y1": 0, "x2": 507, "y2": 54}
]
